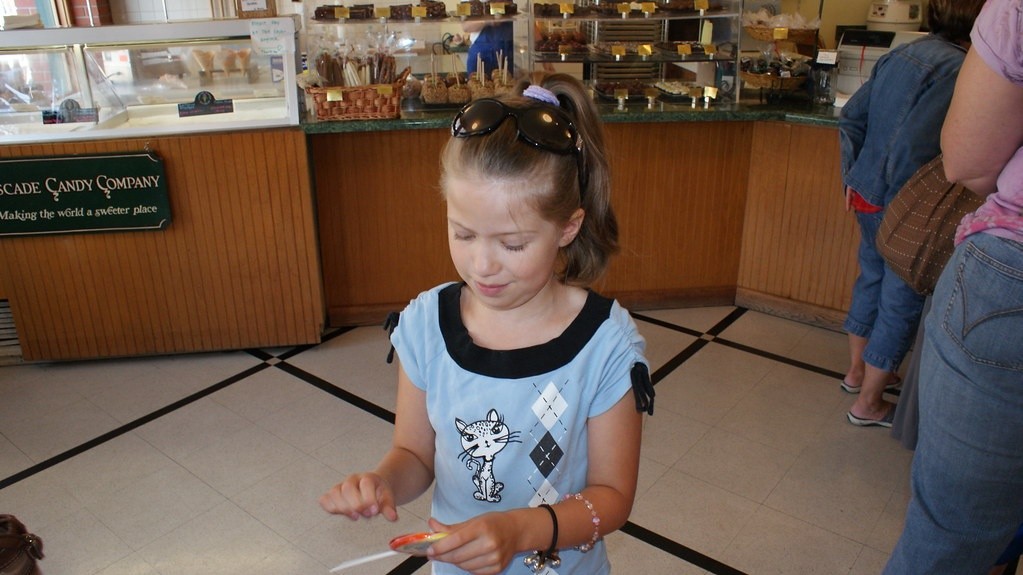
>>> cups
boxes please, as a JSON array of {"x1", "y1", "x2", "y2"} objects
[{"x1": 813, "y1": 68, "x2": 836, "y2": 104}]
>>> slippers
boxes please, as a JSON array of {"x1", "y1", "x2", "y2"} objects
[
  {"x1": 848, "y1": 403, "x2": 896, "y2": 428},
  {"x1": 840, "y1": 370, "x2": 903, "y2": 394}
]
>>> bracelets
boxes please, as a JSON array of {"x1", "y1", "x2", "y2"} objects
[
  {"x1": 563, "y1": 492, "x2": 600, "y2": 553},
  {"x1": 523, "y1": 503, "x2": 561, "y2": 573}
]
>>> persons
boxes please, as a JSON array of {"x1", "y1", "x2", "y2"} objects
[
  {"x1": 460, "y1": 21, "x2": 514, "y2": 73},
  {"x1": 318, "y1": 72, "x2": 657, "y2": 575},
  {"x1": 881, "y1": 0, "x2": 1023, "y2": 575},
  {"x1": 835, "y1": 0, "x2": 987, "y2": 428}
]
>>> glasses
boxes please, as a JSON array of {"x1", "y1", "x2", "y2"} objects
[{"x1": 450, "y1": 97, "x2": 587, "y2": 207}]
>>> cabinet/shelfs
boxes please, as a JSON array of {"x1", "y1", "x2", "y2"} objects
[{"x1": 514, "y1": 1, "x2": 742, "y2": 104}]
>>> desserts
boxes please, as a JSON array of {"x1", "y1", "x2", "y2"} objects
[
  {"x1": 398, "y1": 48, "x2": 518, "y2": 105},
  {"x1": 531, "y1": 2, "x2": 590, "y2": 52},
  {"x1": 315, "y1": 0, "x2": 516, "y2": 19}
]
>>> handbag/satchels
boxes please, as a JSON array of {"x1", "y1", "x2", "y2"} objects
[{"x1": 875, "y1": 150, "x2": 993, "y2": 297}]
